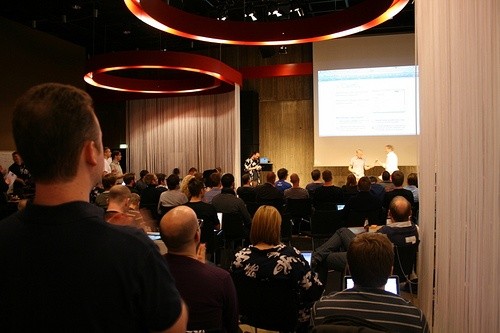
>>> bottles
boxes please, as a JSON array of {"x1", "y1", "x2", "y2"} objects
[
  {"x1": 364, "y1": 218, "x2": 369, "y2": 233},
  {"x1": 386, "y1": 209, "x2": 392, "y2": 225}
]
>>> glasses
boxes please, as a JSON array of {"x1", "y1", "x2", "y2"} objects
[
  {"x1": 202, "y1": 186, "x2": 207, "y2": 189},
  {"x1": 194, "y1": 219, "x2": 204, "y2": 240}
]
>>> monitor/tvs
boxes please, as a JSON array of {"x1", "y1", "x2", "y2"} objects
[
  {"x1": 299, "y1": 250, "x2": 313, "y2": 267},
  {"x1": 343, "y1": 276, "x2": 400, "y2": 294},
  {"x1": 147, "y1": 231, "x2": 160, "y2": 240}
]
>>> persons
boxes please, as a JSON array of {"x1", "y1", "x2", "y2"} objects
[
  {"x1": 160, "y1": 205, "x2": 242, "y2": 333},
  {"x1": 186, "y1": 178, "x2": 220, "y2": 234},
  {"x1": 237, "y1": 174, "x2": 254, "y2": 205},
  {"x1": 212, "y1": 174, "x2": 251, "y2": 248},
  {"x1": 312, "y1": 196, "x2": 419, "y2": 283},
  {"x1": 275, "y1": 168, "x2": 291, "y2": 191},
  {"x1": 103, "y1": 146, "x2": 126, "y2": 184},
  {"x1": 284, "y1": 145, "x2": 419, "y2": 201},
  {"x1": 0, "y1": 152, "x2": 30, "y2": 200},
  {"x1": 309, "y1": 233, "x2": 430, "y2": 332},
  {"x1": 124, "y1": 167, "x2": 197, "y2": 212},
  {"x1": 255, "y1": 172, "x2": 281, "y2": 206},
  {"x1": 126, "y1": 193, "x2": 144, "y2": 228},
  {"x1": 196, "y1": 167, "x2": 222, "y2": 204},
  {"x1": 229, "y1": 205, "x2": 325, "y2": 321},
  {"x1": 158, "y1": 175, "x2": 189, "y2": 213},
  {"x1": 97, "y1": 174, "x2": 116, "y2": 205},
  {"x1": 244, "y1": 152, "x2": 262, "y2": 187},
  {"x1": 106, "y1": 184, "x2": 132, "y2": 226},
  {"x1": 0, "y1": 83, "x2": 187, "y2": 333}
]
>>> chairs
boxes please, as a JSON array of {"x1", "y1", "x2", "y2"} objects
[{"x1": 160, "y1": 191, "x2": 421, "y2": 333}]
